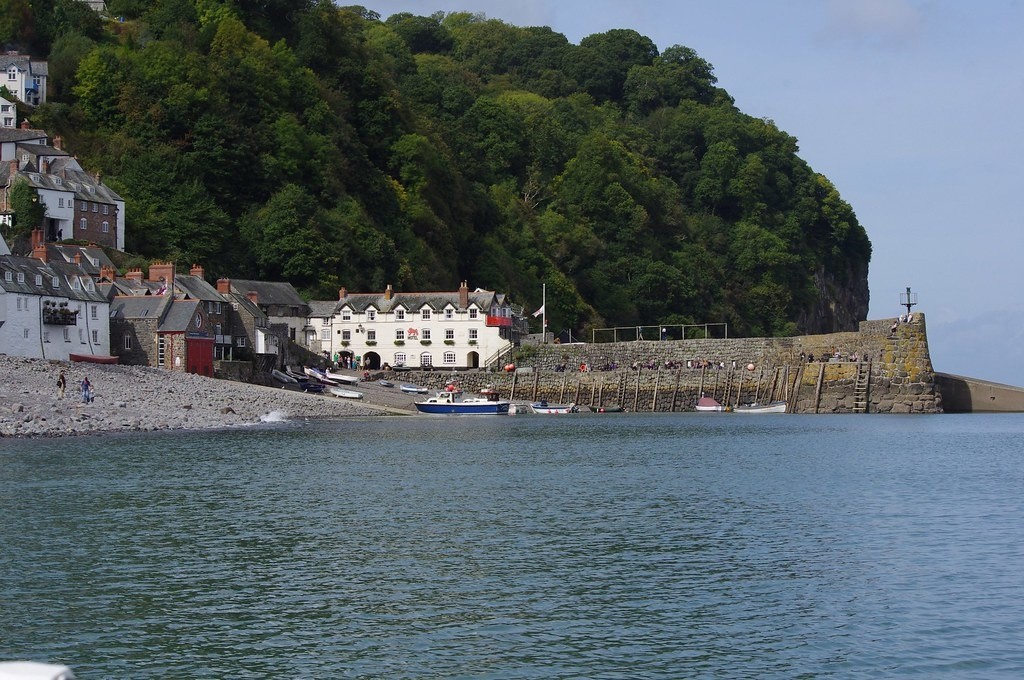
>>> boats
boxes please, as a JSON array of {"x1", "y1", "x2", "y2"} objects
[
  {"x1": 285, "y1": 361, "x2": 359, "y2": 386},
  {"x1": 463, "y1": 388, "x2": 528, "y2": 415},
  {"x1": 330, "y1": 387, "x2": 364, "y2": 399},
  {"x1": 414, "y1": 385, "x2": 511, "y2": 415},
  {"x1": 378, "y1": 379, "x2": 395, "y2": 387},
  {"x1": 695, "y1": 392, "x2": 734, "y2": 412},
  {"x1": 399, "y1": 383, "x2": 428, "y2": 394},
  {"x1": 271, "y1": 369, "x2": 297, "y2": 384},
  {"x1": 298, "y1": 381, "x2": 324, "y2": 392},
  {"x1": 589, "y1": 402, "x2": 626, "y2": 413},
  {"x1": 733, "y1": 401, "x2": 787, "y2": 413},
  {"x1": 530, "y1": 398, "x2": 580, "y2": 414}
]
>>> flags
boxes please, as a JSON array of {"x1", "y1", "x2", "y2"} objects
[
  {"x1": 153, "y1": 284, "x2": 165, "y2": 296},
  {"x1": 531, "y1": 304, "x2": 543, "y2": 318}
]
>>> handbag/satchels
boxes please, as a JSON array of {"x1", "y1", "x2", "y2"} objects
[{"x1": 56, "y1": 381, "x2": 61, "y2": 386}]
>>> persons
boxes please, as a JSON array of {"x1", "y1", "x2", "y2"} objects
[
  {"x1": 56, "y1": 229, "x2": 62, "y2": 241},
  {"x1": 80, "y1": 377, "x2": 92, "y2": 402},
  {"x1": 661, "y1": 328, "x2": 667, "y2": 340},
  {"x1": 638, "y1": 327, "x2": 643, "y2": 341},
  {"x1": 58, "y1": 370, "x2": 66, "y2": 399},
  {"x1": 443, "y1": 385, "x2": 458, "y2": 392},
  {"x1": 316, "y1": 350, "x2": 370, "y2": 380},
  {"x1": 554, "y1": 357, "x2": 725, "y2": 373},
  {"x1": 798, "y1": 350, "x2": 830, "y2": 363},
  {"x1": 890, "y1": 312, "x2": 912, "y2": 332}
]
